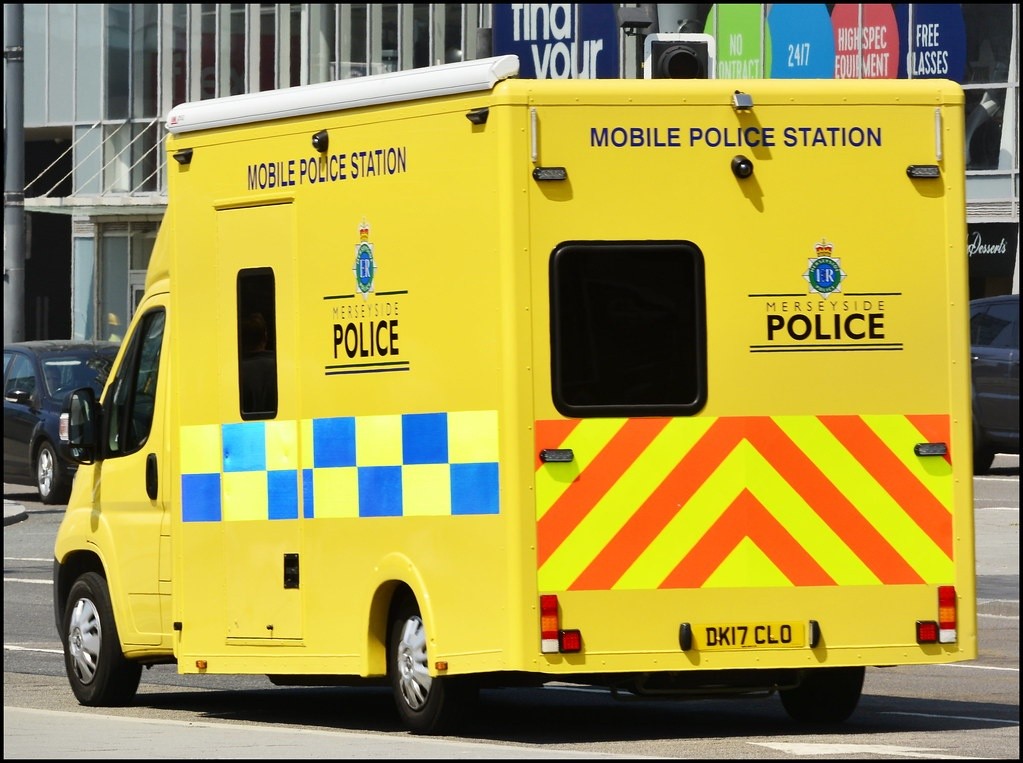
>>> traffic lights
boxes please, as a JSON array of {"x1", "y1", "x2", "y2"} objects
[{"x1": 643, "y1": 33, "x2": 717, "y2": 79}]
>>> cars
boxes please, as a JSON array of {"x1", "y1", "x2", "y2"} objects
[
  {"x1": 2, "y1": 340, "x2": 121, "y2": 505},
  {"x1": 969, "y1": 294, "x2": 1020, "y2": 475}
]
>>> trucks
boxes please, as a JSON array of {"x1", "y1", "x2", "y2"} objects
[{"x1": 53, "y1": 56, "x2": 977, "y2": 732}]
